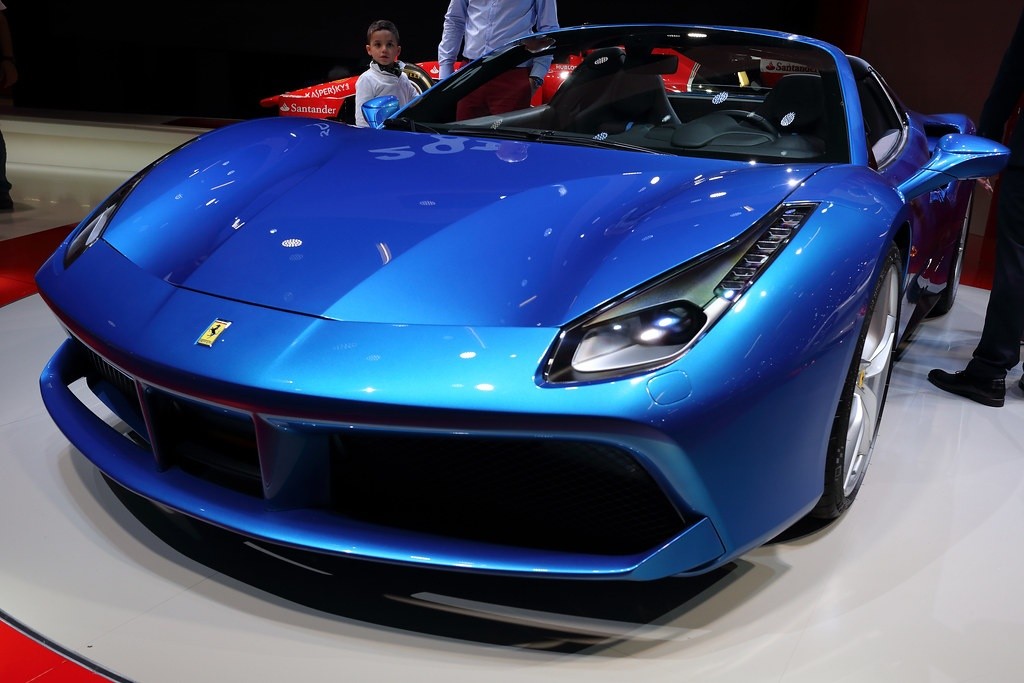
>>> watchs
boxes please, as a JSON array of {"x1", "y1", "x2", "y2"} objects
[{"x1": 532, "y1": 76, "x2": 543, "y2": 87}]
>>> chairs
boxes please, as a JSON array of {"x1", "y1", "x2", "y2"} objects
[
  {"x1": 532, "y1": 47, "x2": 681, "y2": 137},
  {"x1": 737, "y1": 72, "x2": 843, "y2": 143}
]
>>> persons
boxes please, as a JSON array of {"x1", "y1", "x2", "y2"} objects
[
  {"x1": 438, "y1": 0, "x2": 560, "y2": 122},
  {"x1": 355, "y1": 20, "x2": 419, "y2": 127},
  {"x1": 0, "y1": 11, "x2": 19, "y2": 209},
  {"x1": 927, "y1": 8, "x2": 1024, "y2": 408}
]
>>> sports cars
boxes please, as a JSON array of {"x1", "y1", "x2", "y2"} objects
[{"x1": 35, "y1": 21, "x2": 1010, "y2": 657}]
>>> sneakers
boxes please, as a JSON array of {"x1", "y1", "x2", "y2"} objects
[{"x1": 927, "y1": 355, "x2": 1005, "y2": 407}]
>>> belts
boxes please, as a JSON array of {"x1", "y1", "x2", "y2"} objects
[{"x1": 463, "y1": 56, "x2": 474, "y2": 63}]
